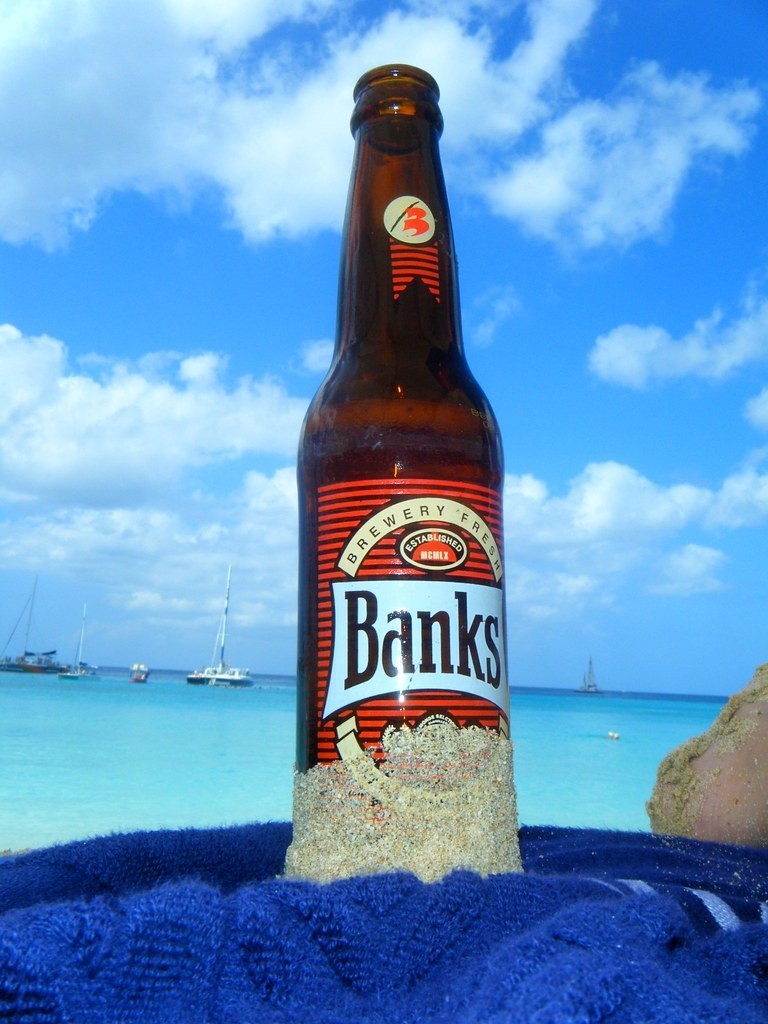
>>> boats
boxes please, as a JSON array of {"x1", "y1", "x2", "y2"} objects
[{"x1": 129, "y1": 664, "x2": 151, "y2": 683}]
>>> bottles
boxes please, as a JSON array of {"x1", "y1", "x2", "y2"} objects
[{"x1": 282, "y1": 64, "x2": 532, "y2": 884}]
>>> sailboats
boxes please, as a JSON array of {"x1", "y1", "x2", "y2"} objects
[
  {"x1": 574, "y1": 658, "x2": 604, "y2": 695},
  {"x1": 186, "y1": 565, "x2": 255, "y2": 688},
  {"x1": 1, "y1": 576, "x2": 68, "y2": 675},
  {"x1": 59, "y1": 602, "x2": 99, "y2": 681}
]
